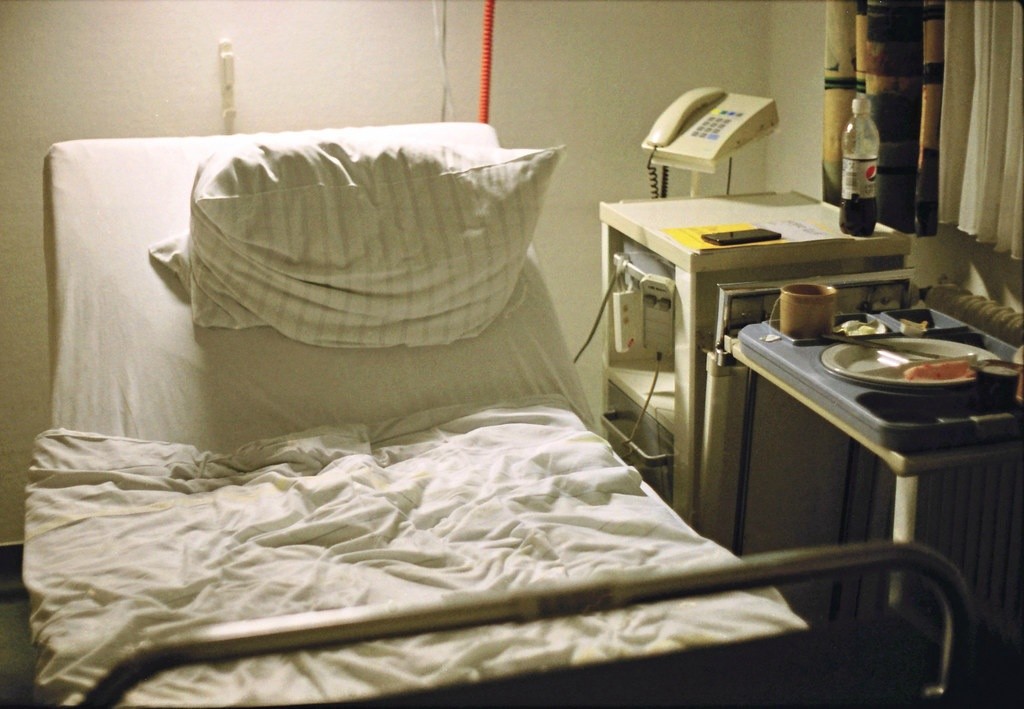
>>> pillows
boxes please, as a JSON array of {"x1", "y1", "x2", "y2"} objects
[{"x1": 146, "y1": 125, "x2": 568, "y2": 352}]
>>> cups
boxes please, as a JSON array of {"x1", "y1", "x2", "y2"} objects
[{"x1": 778, "y1": 284, "x2": 836, "y2": 341}]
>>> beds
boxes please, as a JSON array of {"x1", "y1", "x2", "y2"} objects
[{"x1": 21, "y1": 123, "x2": 813, "y2": 708}]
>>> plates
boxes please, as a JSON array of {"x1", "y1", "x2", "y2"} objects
[{"x1": 820, "y1": 337, "x2": 1002, "y2": 392}]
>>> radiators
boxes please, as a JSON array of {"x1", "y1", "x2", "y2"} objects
[{"x1": 906, "y1": 282, "x2": 1023, "y2": 648}]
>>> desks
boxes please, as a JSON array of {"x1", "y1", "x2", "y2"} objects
[{"x1": 730, "y1": 344, "x2": 1023, "y2": 709}]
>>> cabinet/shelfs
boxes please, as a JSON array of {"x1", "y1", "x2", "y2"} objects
[{"x1": 598, "y1": 188, "x2": 914, "y2": 622}]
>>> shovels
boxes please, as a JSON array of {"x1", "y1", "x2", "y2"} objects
[{"x1": 822, "y1": 332, "x2": 1024, "y2": 377}]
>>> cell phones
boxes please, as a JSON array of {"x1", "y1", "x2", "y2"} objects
[{"x1": 702, "y1": 229, "x2": 782, "y2": 246}]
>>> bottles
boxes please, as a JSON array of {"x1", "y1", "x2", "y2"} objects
[{"x1": 839, "y1": 98, "x2": 881, "y2": 237}]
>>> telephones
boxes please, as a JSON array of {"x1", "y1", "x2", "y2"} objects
[{"x1": 641, "y1": 87, "x2": 779, "y2": 174}]
